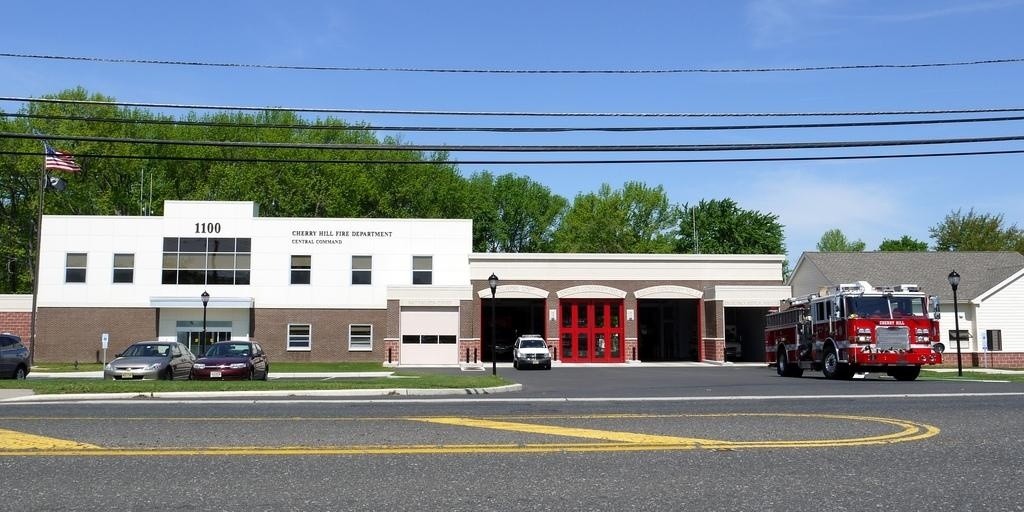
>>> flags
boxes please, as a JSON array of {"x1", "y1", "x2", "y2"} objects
[
  {"x1": 43, "y1": 142, "x2": 82, "y2": 173},
  {"x1": 43, "y1": 176, "x2": 68, "y2": 192}
]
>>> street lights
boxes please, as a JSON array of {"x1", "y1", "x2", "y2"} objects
[
  {"x1": 201, "y1": 292, "x2": 209, "y2": 357},
  {"x1": 948, "y1": 270, "x2": 962, "y2": 376},
  {"x1": 488, "y1": 272, "x2": 498, "y2": 376}
]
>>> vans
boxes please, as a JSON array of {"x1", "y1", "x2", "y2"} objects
[{"x1": 725, "y1": 332, "x2": 742, "y2": 360}]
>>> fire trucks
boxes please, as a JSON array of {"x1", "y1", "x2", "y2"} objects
[{"x1": 764, "y1": 280, "x2": 945, "y2": 380}]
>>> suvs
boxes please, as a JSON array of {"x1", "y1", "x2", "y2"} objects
[{"x1": 513, "y1": 335, "x2": 551, "y2": 369}]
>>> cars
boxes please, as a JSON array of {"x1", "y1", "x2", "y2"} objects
[
  {"x1": 105, "y1": 341, "x2": 196, "y2": 380},
  {"x1": 189, "y1": 341, "x2": 269, "y2": 380},
  {"x1": 0, "y1": 334, "x2": 31, "y2": 379},
  {"x1": 487, "y1": 340, "x2": 513, "y2": 357}
]
